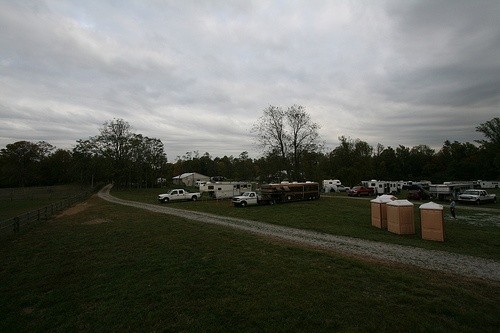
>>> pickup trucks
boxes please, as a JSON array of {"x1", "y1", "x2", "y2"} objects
[
  {"x1": 348, "y1": 185, "x2": 374, "y2": 197},
  {"x1": 458, "y1": 189, "x2": 497, "y2": 203},
  {"x1": 159, "y1": 189, "x2": 200, "y2": 202}
]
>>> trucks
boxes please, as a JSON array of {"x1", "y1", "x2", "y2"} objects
[{"x1": 233, "y1": 182, "x2": 320, "y2": 207}]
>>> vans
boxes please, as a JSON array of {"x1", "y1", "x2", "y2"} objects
[{"x1": 322, "y1": 180, "x2": 350, "y2": 191}]
